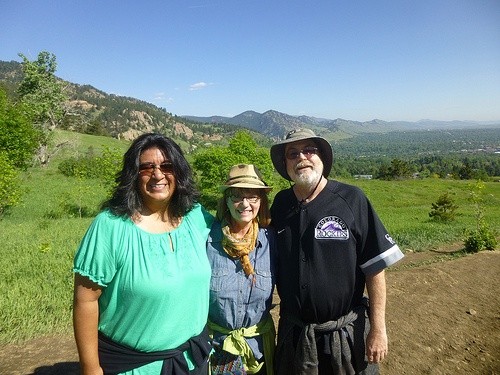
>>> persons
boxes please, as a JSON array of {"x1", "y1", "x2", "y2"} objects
[
  {"x1": 72, "y1": 132, "x2": 216, "y2": 375},
  {"x1": 206, "y1": 128, "x2": 405, "y2": 375}
]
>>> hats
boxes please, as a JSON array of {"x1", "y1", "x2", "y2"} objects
[
  {"x1": 218, "y1": 163, "x2": 274, "y2": 195},
  {"x1": 269, "y1": 128, "x2": 333, "y2": 182}
]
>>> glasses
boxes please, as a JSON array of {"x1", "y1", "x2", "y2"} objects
[
  {"x1": 137, "y1": 159, "x2": 176, "y2": 176},
  {"x1": 227, "y1": 193, "x2": 263, "y2": 204},
  {"x1": 282, "y1": 143, "x2": 323, "y2": 161}
]
